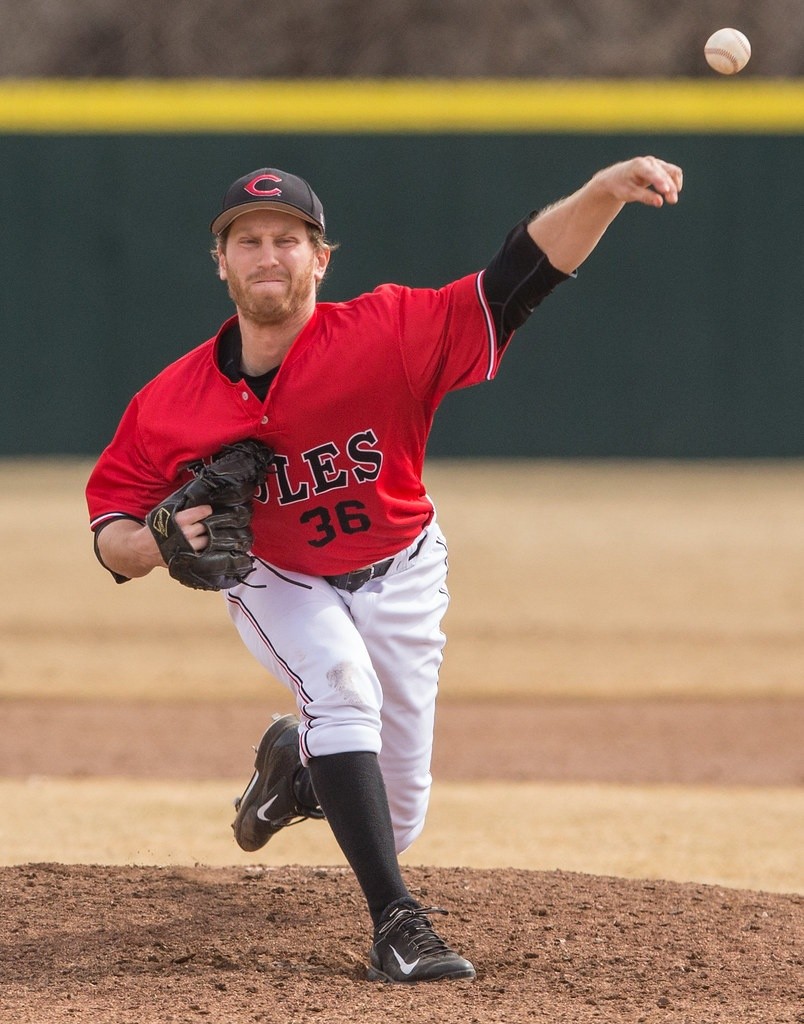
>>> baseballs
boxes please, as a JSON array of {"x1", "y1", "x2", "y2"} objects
[{"x1": 704, "y1": 26, "x2": 753, "y2": 75}]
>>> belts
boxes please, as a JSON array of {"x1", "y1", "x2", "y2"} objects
[{"x1": 323, "y1": 536, "x2": 428, "y2": 593}]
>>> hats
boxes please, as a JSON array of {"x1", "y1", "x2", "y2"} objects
[{"x1": 210, "y1": 168, "x2": 325, "y2": 237}]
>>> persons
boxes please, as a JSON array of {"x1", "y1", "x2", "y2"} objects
[{"x1": 87, "y1": 155, "x2": 685, "y2": 982}]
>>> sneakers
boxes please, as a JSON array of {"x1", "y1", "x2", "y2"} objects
[
  {"x1": 367, "y1": 897, "x2": 476, "y2": 984},
  {"x1": 230, "y1": 713, "x2": 325, "y2": 852}
]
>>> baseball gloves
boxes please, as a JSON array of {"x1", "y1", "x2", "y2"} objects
[{"x1": 143, "y1": 437, "x2": 274, "y2": 593}]
